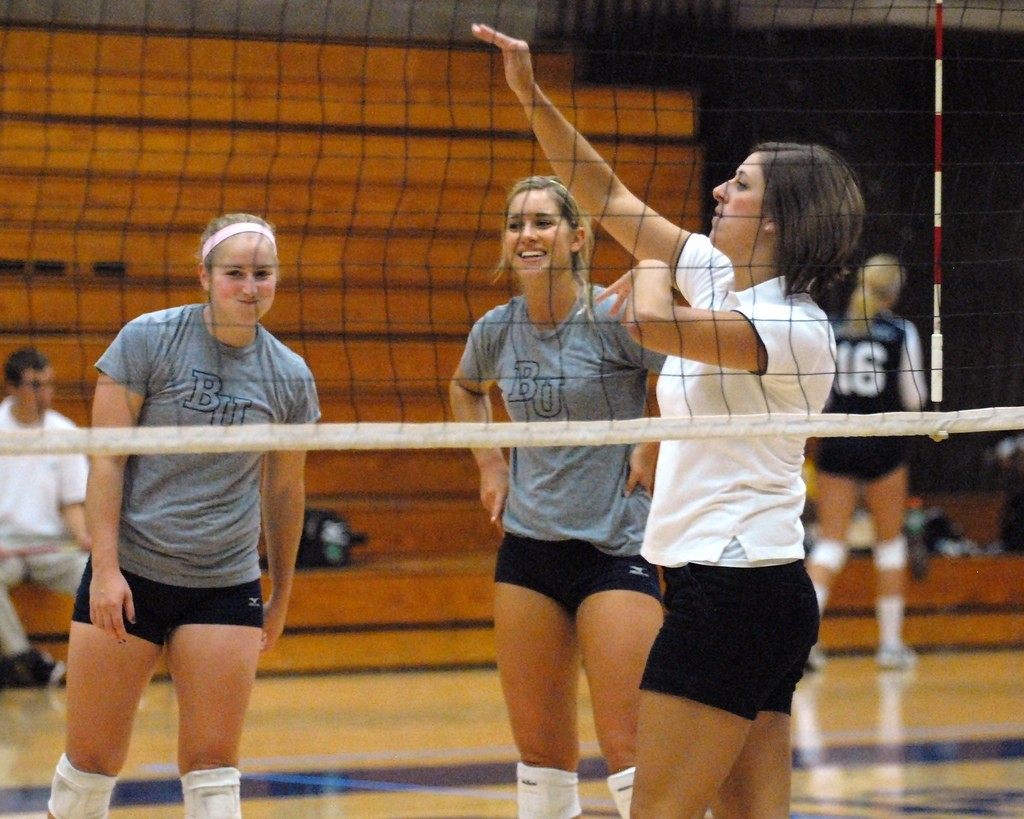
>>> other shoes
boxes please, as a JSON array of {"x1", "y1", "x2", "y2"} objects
[
  {"x1": 874, "y1": 642, "x2": 916, "y2": 671},
  {"x1": 9, "y1": 653, "x2": 48, "y2": 686},
  {"x1": 806, "y1": 647, "x2": 820, "y2": 673}
]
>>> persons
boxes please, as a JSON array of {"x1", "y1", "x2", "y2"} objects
[
  {"x1": 471, "y1": 23, "x2": 867, "y2": 819},
  {"x1": 449, "y1": 174, "x2": 670, "y2": 819},
  {"x1": 804, "y1": 251, "x2": 928, "y2": 671},
  {"x1": 47, "y1": 212, "x2": 322, "y2": 819},
  {"x1": 0, "y1": 344, "x2": 93, "y2": 687}
]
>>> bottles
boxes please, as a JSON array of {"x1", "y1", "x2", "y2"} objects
[{"x1": 901, "y1": 495, "x2": 927, "y2": 555}]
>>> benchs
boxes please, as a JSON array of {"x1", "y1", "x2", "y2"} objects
[{"x1": 0, "y1": 23, "x2": 1024, "y2": 650}]
